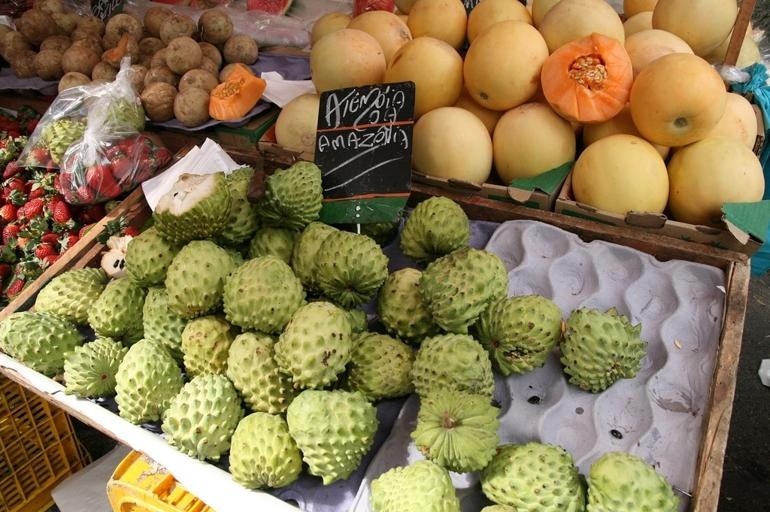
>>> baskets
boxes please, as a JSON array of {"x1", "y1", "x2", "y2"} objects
[
  {"x1": 0, "y1": 379, "x2": 93, "y2": 512},
  {"x1": 106, "y1": 449, "x2": 216, "y2": 512}
]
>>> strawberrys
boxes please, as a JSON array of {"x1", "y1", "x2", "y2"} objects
[{"x1": 0, "y1": 113, "x2": 174, "y2": 300}]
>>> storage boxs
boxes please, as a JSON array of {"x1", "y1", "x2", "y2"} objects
[{"x1": 0, "y1": 90, "x2": 762, "y2": 512}]
